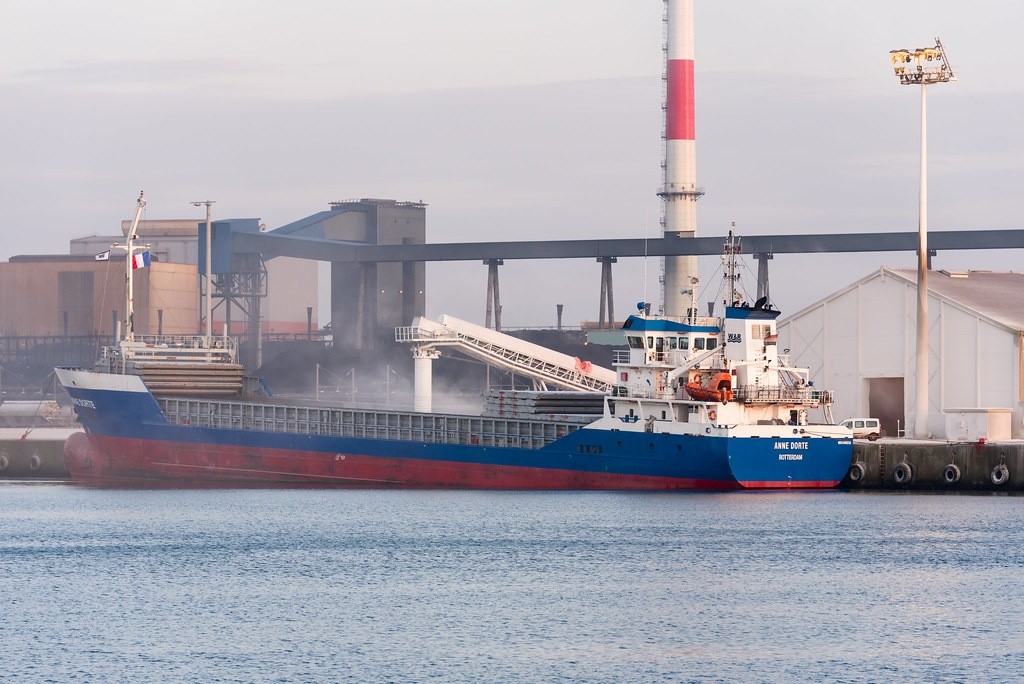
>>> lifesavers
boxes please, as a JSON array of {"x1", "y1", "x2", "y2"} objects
[
  {"x1": 0, "y1": 455, "x2": 8, "y2": 470},
  {"x1": 944, "y1": 463, "x2": 961, "y2": 482},
  {"x1": 892, "y1": 463, "x2": 912, "y2": 484},
  {"x1": 215, "y1": 341, "x2": 223, "y2": 349},
  {"x1": 693, "y1": 374, "x2": 702, "y2": 384},
  {"x1": 848, "y1": 464, "x2": 865, "y2": 483},
  {"x1": 708, "y1": 410, "x2": 716, "y2": 420},
  {"x1": 29, "y1": 455, "x2": 41, "y2": 471},
  {"x1": 991, "y1": 465, "x2": 1010, "y2": 485}
]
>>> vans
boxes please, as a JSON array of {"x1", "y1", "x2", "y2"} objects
[{"x1": 836, "y1": 417, "x2": 882, "y2": 442}]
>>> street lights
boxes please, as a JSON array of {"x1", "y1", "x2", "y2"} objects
[{"x1": 891, "y1": 35, "x2": 956, "y2": 442}]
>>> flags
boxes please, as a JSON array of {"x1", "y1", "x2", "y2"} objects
[
  {"x1": 132, "y1": 251, "x2": 151, "y2": 270},
  {"x1": 95, "y1": 250, "x2": 111, "y2": 261}
]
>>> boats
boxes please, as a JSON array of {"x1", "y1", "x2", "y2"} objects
[
  {"x1": 91, "y1": 188, "x2": 247, "y2": 401},
  {"x1": 684, "y1": 372, "x2": 734, "y2": 402},
  {"x1": 53, "y1": 220, "x2": 850, "y2": 493}
]
